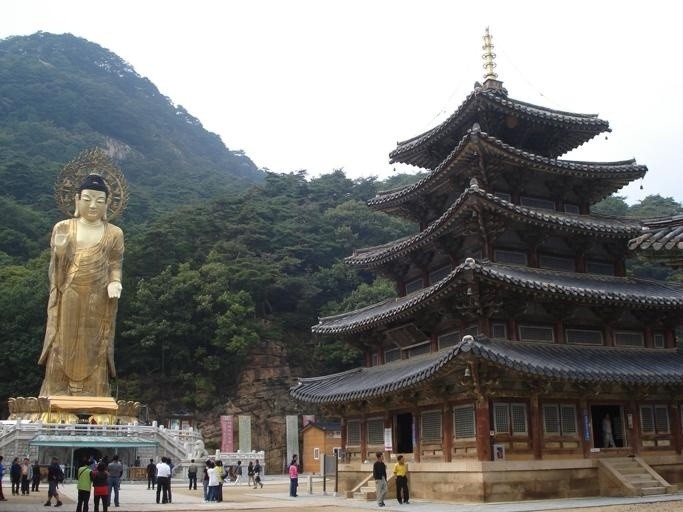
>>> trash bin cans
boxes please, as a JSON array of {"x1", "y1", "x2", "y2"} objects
[{"x1": 491, "y1": 445, "x2": 505, "y2": 460}]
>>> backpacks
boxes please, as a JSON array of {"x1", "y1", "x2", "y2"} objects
[{"x1": 55, "y1": 464, "x2": 65, "y2": 483}]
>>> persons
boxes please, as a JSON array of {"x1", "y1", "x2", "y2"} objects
[
  {"x1": 373, "y1": 452, "x2": 388, "y2": 507},
  {"x1": 116, "y1": 419, "x2": 120, "y2": 430},
  {"x1": 91, "y1": 419, "x2": 97, "y2": 424},
  {"x1": 393, "y1": 456, "x2": 409, "y2": 504},
  {"x1": 601, "y1": 413, "x2": 616, "y2": 448},
  {"x1": 38, "y1": 174, "x2": 125, "y2": 397},
  {"x1": 0, "y1": 454, "x2": 299, "y2": 512}
]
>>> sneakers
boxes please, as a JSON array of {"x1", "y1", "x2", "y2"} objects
[
  {"x1": 12, "y1": 489, "x2": 41, "y2": 496},
  {"x1": 43, "y1": 501, "x2": 52, "y2": 506},
  {"x1": 253, "y1": 483, "x2": 264, "y2": 490},
  {"x1": 54, "y1": 500, "x2": 62, "y2": 507},
  {"x1": 377, "y1": 500, "x2": 386, "y2": 506}
]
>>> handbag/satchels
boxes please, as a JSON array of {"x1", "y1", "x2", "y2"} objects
[{"x1": 253, "y1": 472, "x2": 259, "y2": 480}]
